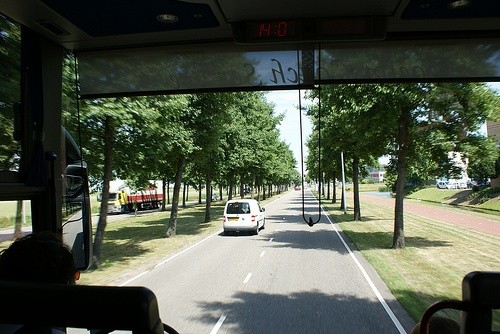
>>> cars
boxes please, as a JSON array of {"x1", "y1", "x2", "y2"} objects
[{"x1": 437, "y1": 176, "x2": 491, "y2": 189}]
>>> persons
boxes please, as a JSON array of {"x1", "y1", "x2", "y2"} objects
[{"x1": 0, "y1": 234, "x2": 76, "y2": 334}]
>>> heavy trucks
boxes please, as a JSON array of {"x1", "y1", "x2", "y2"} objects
[{"x1": 96, "y1": 180, "x2": 164, "y2": 213}]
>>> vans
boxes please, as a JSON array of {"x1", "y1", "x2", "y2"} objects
[{"x1": 223, "y1": 198, "x2": 266, "y2": 236}]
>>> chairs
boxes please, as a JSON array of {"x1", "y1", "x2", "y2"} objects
[{"x1": 0, "y1": 284, "x2": 164, "y2": 334}]
